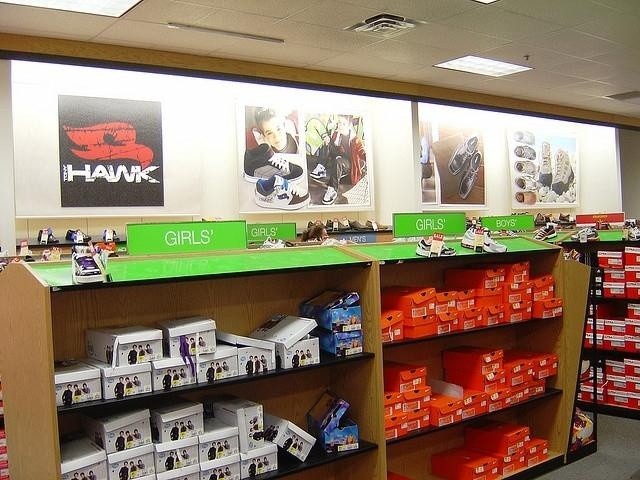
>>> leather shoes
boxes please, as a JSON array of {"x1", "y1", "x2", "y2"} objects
[
  {"x1": 448, "y1": 134, "x2": 479, "y2": 175},
  {"x1": 458, "y1": 151, "x2": 482, "y2": 200}
]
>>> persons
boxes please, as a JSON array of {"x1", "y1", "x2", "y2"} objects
[
  {"x1": 54, "y1": 336, "x2": 312, "y2": 406},
  {"x1": 335, "y1": 316, "x2": 358, "y2": 355},
  {"x1": 347, "y1": 434, "x2": 354, "y2": 444},
  {"x1": 64, "y1": 418, "x2": 303, "y2": 480},
  {"x1": 245, "y1": 106, "x2": 299, "y2": 154},
  {"x1": 309, "y1": 114, "x2": 366, "y2": 205},
  {"x1": 257, "y1": 311, "x2": 288, "y2": 333}
]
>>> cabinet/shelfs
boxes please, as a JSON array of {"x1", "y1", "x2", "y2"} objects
[{"x1": 0, "y1": 232, "x2": 597, "y2": 480}]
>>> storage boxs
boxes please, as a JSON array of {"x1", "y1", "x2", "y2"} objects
[
  {"x1": 380, "y1": 260, "x2": 564, "y2": 480},
  {"x1": 571, "y1": 240, "x2": 640, "y2": 413},
  {"x1": 55, "y1": 288, "x2": 363, "y2": 480}
]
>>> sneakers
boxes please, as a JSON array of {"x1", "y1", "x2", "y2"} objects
[
  {"x1": 322, "y1": 185, "x2": 338, "y2": 204},
  {"x1": 589, "y1": 220, "x2": 609, "y2": 230},
  {"x1": 72, "y1": 245, "x2": 105, "y2": 284},
  {"x1": 621, "y1": 219, "x2": 640, "y2": 241},
  {"x1": 513, "y1": 130, "x2": 579, "y2": 207},
  {"x1": 511, "y1": 210, "x2": 575, "y2": 227},
  {"x1": 257, "y1": 217, "x2": 387, "y2": 250},
  {"x1": 254, "y1": 178, "x2": 311, "y2": 210},
  {"x1": 416, "y1": 237, "x2": 457, "y2": 257},
  {"x1": 534, "y1": 222, "x2": 556, "y2": 241},
  {"x1": 243, "y1": 143, "x2": 304, "y2": 182},
  {"x1": 65, "y1": 229, "x2": 93, "y2": 242},
  {"x1": 103, "y1": 229, "x2": 117, "y2": 240},
  {"x1": 310, "y1": 165, "x2": 327, "y2": 178},
  {"x1": 38, "y1": 228, "x2": 60, "y2": 243},
  {"x1": 570, "y1": 228, "x2": 601, "y2": 242},
  {"x1": 461, "y1": 226, "x2": 508, "y2": 255}
]
